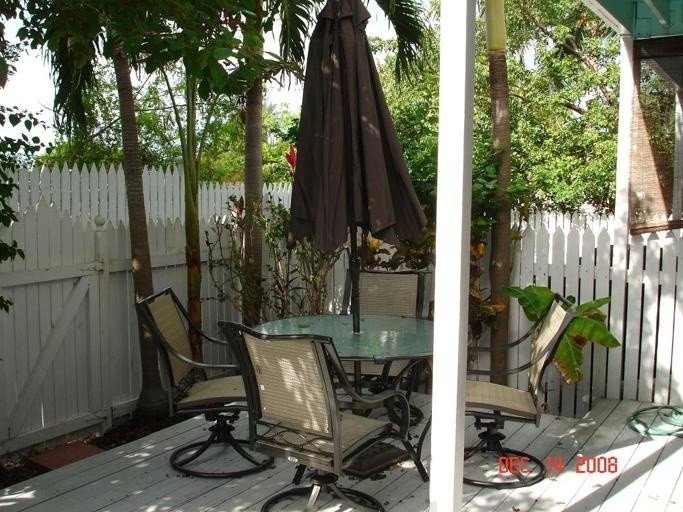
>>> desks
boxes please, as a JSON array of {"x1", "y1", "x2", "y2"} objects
[{"x1": 250, "y1": 314, "x2": 434, "y2": 486}]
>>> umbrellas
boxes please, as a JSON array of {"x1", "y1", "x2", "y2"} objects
[{"x1": 289, "y1": 1, "x2": 428, "y2": 395}]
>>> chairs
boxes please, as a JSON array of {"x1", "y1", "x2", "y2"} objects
[
  {"x1": 351, "y1": 269, "x2": 426, "y2": 428},
  {"x1": 215, "y1": 320, "x2": 411, "y2": 512},
  {"x1": 135, "y1": 286, "x2": 275, "y2": 480},
  {"x1": 462, "y1": 292, "x2": 579, "y2": 490}
]
